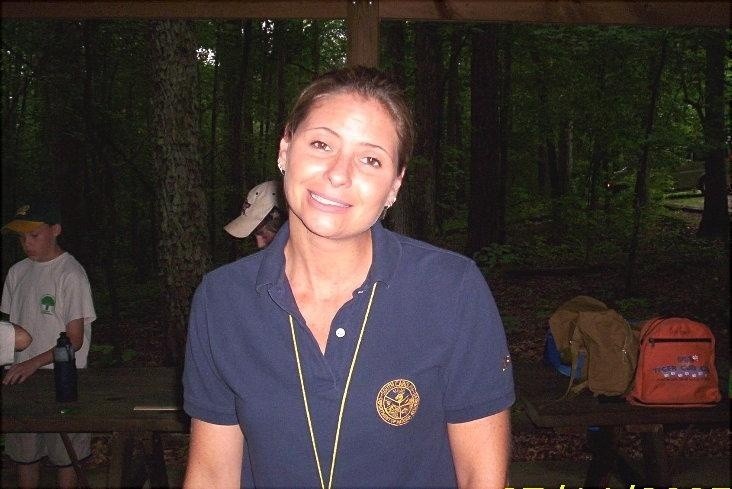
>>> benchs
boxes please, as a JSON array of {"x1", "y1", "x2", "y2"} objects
[{"x1": 524, "y1": 396, "x2": 732, "y2": 489}]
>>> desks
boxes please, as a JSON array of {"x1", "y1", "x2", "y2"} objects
[{"x1": 0, "y1": 367, "x2": 194, "y2": 489}]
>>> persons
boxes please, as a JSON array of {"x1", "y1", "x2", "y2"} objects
[
  {"x1": 0, "y1": 193, "x2": 99, "y2": 487},
  {"x1": 220, "y1": 181, "x2": 286, "y2": 246},
  {"x1": 0, "y1": 312, "x2": 34, "y2": 368},
  {"x1": 170, "y1": 64, "x2": 518, "y2": 488}
]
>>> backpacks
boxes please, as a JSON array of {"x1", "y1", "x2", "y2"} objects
[{"x1": 626, "y1": 314, "x2": 724, "y2": 409}]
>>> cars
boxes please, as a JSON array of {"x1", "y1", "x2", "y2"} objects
[{"x1": 604, "y1": 150, "x2": 705, "y2": 196}]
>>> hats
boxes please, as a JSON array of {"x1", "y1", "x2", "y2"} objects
[
  {"x1": 1, "y1": 203, "x2": 62, "y2": 236},
  {"x1": 222, "y1": 180, "x2": 283, "y2": 240}
]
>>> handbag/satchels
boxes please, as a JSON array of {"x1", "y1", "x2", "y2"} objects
[{"x1": 545, "y1": 294, "x2": 637, "y2": 401}]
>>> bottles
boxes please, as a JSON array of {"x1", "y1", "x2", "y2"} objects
[{"x1": 53, "y1": 332, "x2": 78, "y2": 403}]
